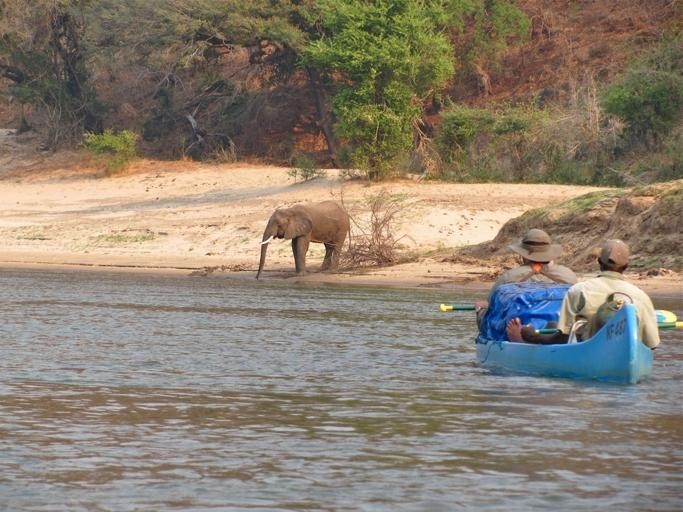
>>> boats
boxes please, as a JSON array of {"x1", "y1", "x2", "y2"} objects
[{"x1": 475, "y1": 303, "x2": 654, "y2": 385}]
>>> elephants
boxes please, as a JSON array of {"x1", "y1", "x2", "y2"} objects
[{"x1": 255, "y1": 198, "x2": 349, "y2": 281}]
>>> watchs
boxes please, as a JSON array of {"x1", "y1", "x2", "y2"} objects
[{"x1": 476, "y1": 306, "x2": 487, "y2": 316}]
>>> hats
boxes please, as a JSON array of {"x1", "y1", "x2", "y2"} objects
[
  {"x1": 599, "y1": 238, "x2": 630, "y2": 269},
  {"x1": 510, "y1": 228, "x2": 563, "y2": 264}
]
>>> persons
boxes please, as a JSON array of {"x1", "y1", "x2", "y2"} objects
[
  {"x1": 475, "y1": 228, "x2": 578, "y2": 333},
  {"x1": 505, "y1": 239, "x2": 660, "y2": 344}
]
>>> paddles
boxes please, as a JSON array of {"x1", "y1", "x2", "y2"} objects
[{"x1": 439, "y1": 304, "x2": 676, "y2": 324}]
